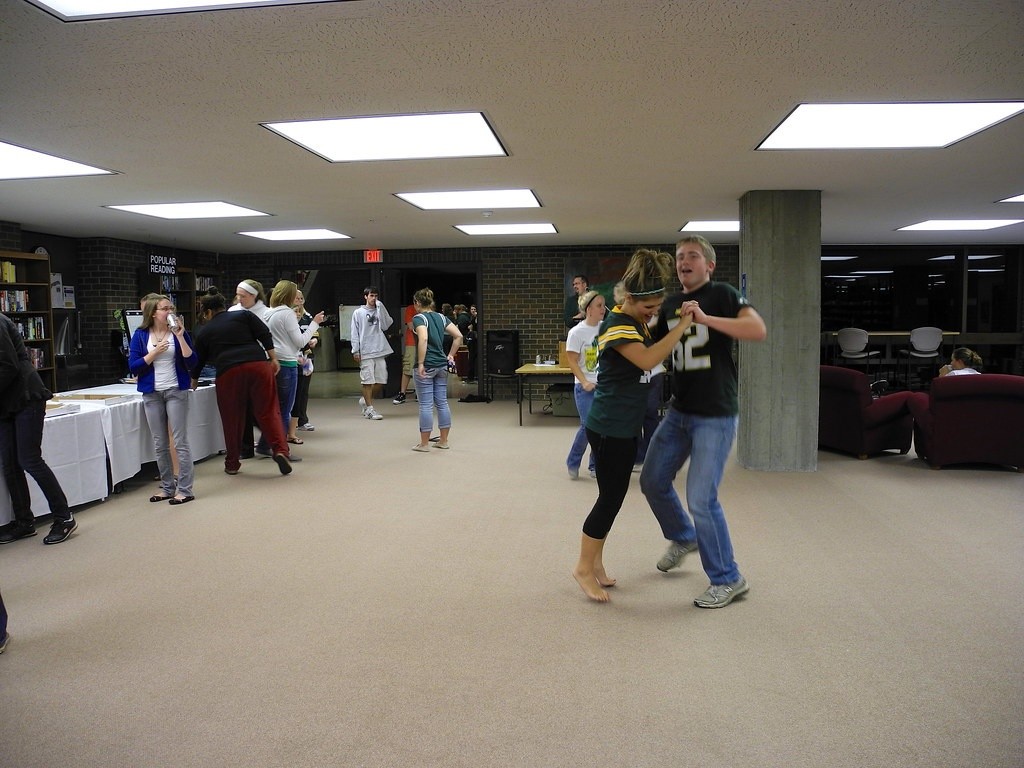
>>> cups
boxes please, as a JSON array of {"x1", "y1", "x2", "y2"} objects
[
  {"x1": 167, "y1": 314, "x2": 179, "y2": 332},
  {"x1": 536, "y1": 355, "x2": 541, "y2": 364}
]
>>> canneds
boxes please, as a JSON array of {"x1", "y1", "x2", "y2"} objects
[
  {"x1": 536, "y1": 355, "x2": 540, "y2": 364},
  {"x1": 167, "y1": 314, "x2": 179, "y2": 331}
]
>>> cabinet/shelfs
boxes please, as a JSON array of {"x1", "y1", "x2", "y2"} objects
[
  {"x1": 0, "y1": 251, "x2": 57, "y2": 393},
  {"x1": 136, "y1": 265, "x2": 222, "y2": 346}
]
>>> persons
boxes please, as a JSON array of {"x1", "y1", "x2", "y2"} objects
[
  {"x1": 190, "y1": 286, "x2": 293, "y2": 475},
  {"x1": 287, "y1": 304, "x2": 304, "y2": 444},
  {"x1": 140, "y1": 294, "x2": 179, "y2": 488},
  {"x1": 351, "y1": 285, "x2": 393, "y2": 420},
  {"x1": 296, "y1": 288, "x2": 319, "y2": 431},
  {"x1": 443, "y1": 303, "x2": 478, "y2": 384},
  {"x1": 129, "y1": 293, "x2": 198, "y2": 505},
  {"x1": 227, "y1": 279, "x2": 270, "y2": 460},
  {"x1": 256, "y1": 280, "x2": 324, "y2": 461},
  {"x1": 573, "y1": 236, "x2": 767, "y2": 609},
  {"x1": 191, "y1": 312, "x2": 216, "y2": 378},
  {"x1": 0, "y1": 313, "x2": 79, "y2": 545},
  {"x1": 564, "y1": 276, "x2": 610, "y2": 479},
  {"x1": 392, "y1": 287, "x2": 463, "y2": 451},
  {"x1": 939, "y1": 347, "x2": 982, "y2": 376}
]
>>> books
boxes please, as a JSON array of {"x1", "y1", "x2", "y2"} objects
[
  {"x1": 0, "y1": 261, "x2": 29, "y2": 311},
  {"x1": 166, "y1": 294, "x2": 176, "y2": 305},
  {"x1": 197, "y1": 277, "x2": 213, "y2": 290},
  {"x1": 9, "y1": 317, "x2": 44, "y2": 340},
  {"x1": 162, "y1": 275, "x2": 178, "y2": 290},
  {"x1": 26, "y1": 346, "x2": 44, "y2": 369}
]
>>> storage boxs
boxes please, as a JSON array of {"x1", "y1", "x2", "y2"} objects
[
  {"x1": 50, "y1": 272, "x2": 76, "y2": 308},
  {"x1": 558, "y1": 340, "x2": 571, "y2": 367},
  {"x1": 547, "y1": 383, "x2": 579, "y2": 416}
]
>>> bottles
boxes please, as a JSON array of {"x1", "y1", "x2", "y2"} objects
[{"x1": 939, "y1": 365, "x2": 952, "y2": 374}]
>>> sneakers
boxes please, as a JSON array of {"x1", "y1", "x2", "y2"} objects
[
  {"x1": 43, "y1": 512, "x2": 78, "y2": 544},
  {"x1": 657, "y1": 541, "x2": 700, "y2": 572},
  {"x1": 0, "y1": 519, "x2": 37, "y2": 544},
  {"x1": 693, "y1": 575, "x2": 750, "y2": 609},
  {"x1": 364, "y1": 406, "x2": 384, "y2": 420},
  {"x1": 358, "y1": 396, "x2": 367, "y2": 416}
]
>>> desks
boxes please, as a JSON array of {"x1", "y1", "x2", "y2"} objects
[
  {"x1": 515, "y1": 363, "x2": 663, "y2": 425},
  {"x1": 0, "y1": 378, "x2": 262, "y2": 527},
  {"x1": 833, "y1": 332, "x2": 961, "y2": 379}
]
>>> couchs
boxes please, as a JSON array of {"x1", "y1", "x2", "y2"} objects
[
  {"x1": 455, "y1": 346, "x2": 469, "y2": 376},
  {"x1": 819, "y1": 364, "x2": 1024, "y2": 471}
]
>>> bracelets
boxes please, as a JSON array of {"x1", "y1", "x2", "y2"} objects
[{"x1": 418, "y1": 361, "x2": 423, "y2": 364}]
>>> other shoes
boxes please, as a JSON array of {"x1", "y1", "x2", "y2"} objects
[
  {"x1": 568, "y1": 466, "x2": 579, "y2": 480},
  {"x1": 414, "y1": 389, "x2": 419, "y2": 402},
  {"x1": 393, "y1": 392, "x2": 406, "y2": 404},
  {"x1": 288, "y1": 453, "x2": 302, "y2": 462},
  {"x1": 154, "y1": 474, "x2": 179, "y2": 488},
  {"x1": 287, "y1": 436, "x2": 303, "y2": 444},
  {"x1": 590, "y1": 470, "x2": 597, "y2": 479},
  {"x1": 0, "y1": 632, "x2": 10, "y2": 654},
  {"x1": 224, "y1": 468, "x2": 238, "y2": 475},
  {"x1": 255, "y1": 447, "x2": 273, "y2": 458},
  {"x1": 169, "y1": 496, "x2": 195, "y2": 505},
  {"x1": 298, "y1": 422, "x2": 314, "y2": 431},
  {"x1": 150, "y1": 495, "x2": 175, "y2": 502},
  {"x1": 632, "y1": 463, "x2": 646, "y2": 472},
  {"x1": 240, "y1": 452, "x2": 254, "y2": 459},
  {"x1": 274, "y1": 453, "x2": 292, "y2": 475},
  {"x1": 411, "y1": 443, "x2": 430, "y2": 452},
  {"x1": 432, "y1": 442, "x2": 450, "y2": 449}
]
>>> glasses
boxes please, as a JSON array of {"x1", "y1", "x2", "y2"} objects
[{"x1": 154, "y1": 306, "x2": 175, "y2": 312}]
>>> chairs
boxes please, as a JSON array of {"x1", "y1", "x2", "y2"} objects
[
  {"x1": 834, "y1": 327, "x2": 881, "y2": 379},
  {"x1": 897, "y1": 327, "x2": 944, "y2": 385}
]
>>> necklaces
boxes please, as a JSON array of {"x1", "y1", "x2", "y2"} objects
[{"x1": 152, "y1": 328, "x2": 168, "y2": 342}]
